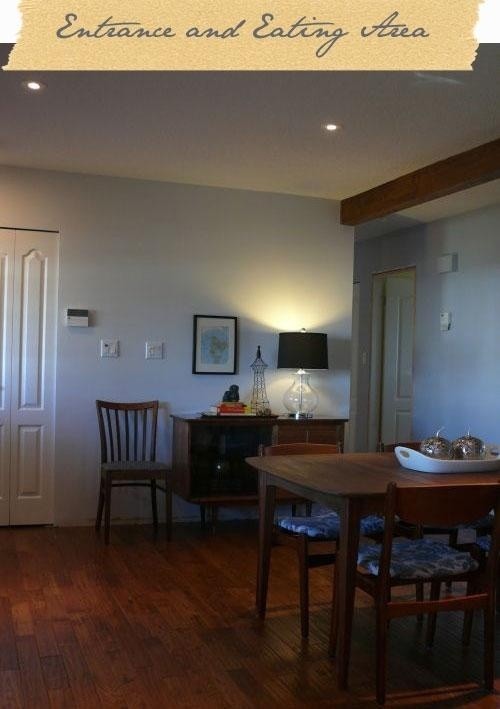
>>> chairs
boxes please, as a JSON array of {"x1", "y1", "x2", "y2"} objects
[
  {"x1": 96, "y1": 399, "x2": 172, "y2": 545},
  {"x1": 259, "y1": 442, "x2": 384, "y2": 635},
  {"x1": 464, "y1": 532, "x2": 500, "y2": 635},
  {"x1": 383, "y1": 441, "x2": 474, "y2": 621},
  {"x1": 355, "y1": 482, "x2": 500, "y2": 704}
]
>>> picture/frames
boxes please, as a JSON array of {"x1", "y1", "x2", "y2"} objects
[{"x1": 192, "y1": 315, "x2": 237, "y2": 375}]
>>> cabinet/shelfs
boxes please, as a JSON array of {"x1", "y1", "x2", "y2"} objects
[
  {"x1": 170, "y1": 414, "x2": 274, "y2": 529},
  {"x1": 272, "y1": 416, "x2": 349, "y2": 518}
]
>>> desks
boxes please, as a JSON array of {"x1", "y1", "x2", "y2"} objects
[{"x1": 246, "y1": 452, "x2": 500, "y2": 692}]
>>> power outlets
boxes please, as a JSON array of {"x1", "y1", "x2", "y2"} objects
[{"x1": 145, "y1": 342, "x2": 163, "y2": 360}]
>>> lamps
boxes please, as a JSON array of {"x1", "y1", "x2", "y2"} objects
[{"x1": 277, "y1": 333, "x2": 328, "y2": 418}]
